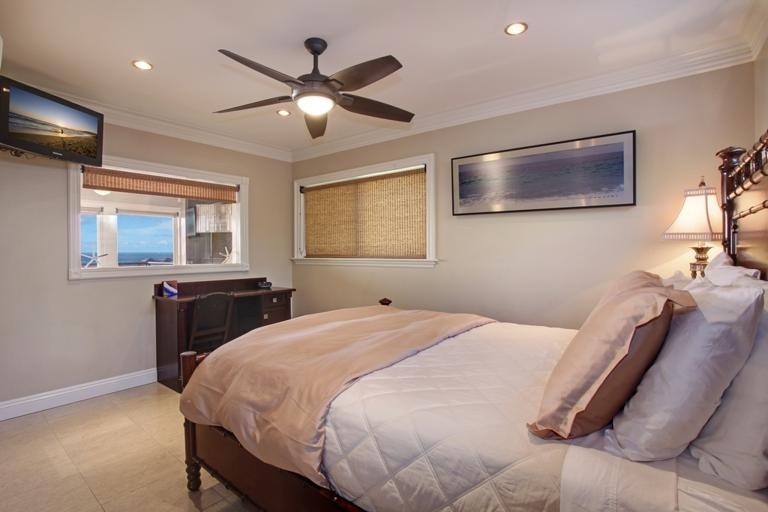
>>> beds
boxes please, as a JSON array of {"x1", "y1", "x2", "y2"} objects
[{"x1": 180, "y1": 127, "x2": 768, "y2": 512}]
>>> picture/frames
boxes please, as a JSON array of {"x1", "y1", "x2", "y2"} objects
[{"x1": 449, "y1": 130, "x2": 636, "y2": 213}]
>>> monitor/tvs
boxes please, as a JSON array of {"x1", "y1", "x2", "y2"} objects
[{"x1": 0, "y1": 75, "x2": 104, "y2": 167}]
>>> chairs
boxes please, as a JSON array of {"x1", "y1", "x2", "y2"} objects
[{"x1": 185, "y1": 289, "x2": 236, "y2": 358}]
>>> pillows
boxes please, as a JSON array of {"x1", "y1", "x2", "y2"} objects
[
  {"x1": 708, "y1": 263, "x2": 761, "y2": 286},
  {"x1": 575, "y1": 273, "x2": 766, "y2": 462},
  {"x1": 684, "y1": 274, "x2": 767, "y2": 490},
  {"x1": 526, "y1": 268, "x2": 699, "y2": 442}
]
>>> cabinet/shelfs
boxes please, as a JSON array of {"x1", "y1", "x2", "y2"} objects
[{"x1": 154, "y1": 277, "x2": 297, "y2": 394}]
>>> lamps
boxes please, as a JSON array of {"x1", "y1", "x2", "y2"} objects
[
  {"x1": 663, "y1": 176, "x2": 727, "y2": 284},
  {"x1": 290, "y1": 39, "x2": 337, "y2": 118}
]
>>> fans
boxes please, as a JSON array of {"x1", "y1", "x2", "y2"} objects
[{"x1": 211, "y1": 38, "x2": 414, "y2": 140}]
